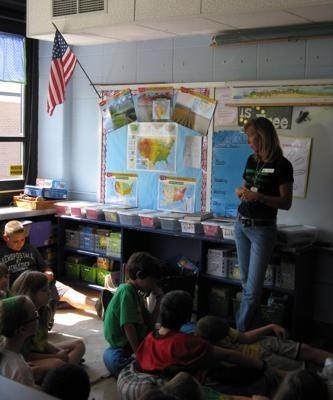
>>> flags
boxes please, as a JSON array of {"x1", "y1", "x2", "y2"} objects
[{"x1": 45, "y1": 28, "x2": 76, "y2": 118}]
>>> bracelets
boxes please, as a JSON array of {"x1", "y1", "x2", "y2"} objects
[{"x1": 261, "y1": 194, "x2": 266, "y2": 204}]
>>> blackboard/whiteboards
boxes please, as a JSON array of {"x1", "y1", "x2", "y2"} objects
[{"x1": 97, "y1": 79, "x2": 332, "y2": 251}]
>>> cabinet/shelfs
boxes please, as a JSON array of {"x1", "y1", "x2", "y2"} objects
[{"x1": 0, "y1": 206, "x2": 318, "y2": 344}]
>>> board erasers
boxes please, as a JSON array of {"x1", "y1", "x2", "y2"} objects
[{"x1": 314, "y1": 241, "x2": 331, "y2": 246}]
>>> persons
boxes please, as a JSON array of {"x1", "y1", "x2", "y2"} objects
[
  {"x1": 0, "y1": 221, "x2": 332, "y2": 399},
  {"x1": 230, "y1": 117, "x2": 295, "y2": 333}
]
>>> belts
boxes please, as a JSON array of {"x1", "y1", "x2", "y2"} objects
[{"x1": 239, "y1": 218, "x2": 275, "y2": 227}]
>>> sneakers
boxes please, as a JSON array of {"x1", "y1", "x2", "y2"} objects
[{"x1": 95, "y1": 289, "x2": 113, "y2": 321}]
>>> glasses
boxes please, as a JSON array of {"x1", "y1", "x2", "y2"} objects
[{"x1": 24, "y1": 311, "x2": 41, "y2": 327}]
